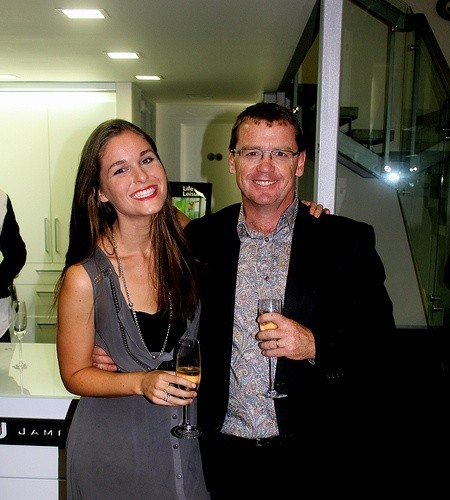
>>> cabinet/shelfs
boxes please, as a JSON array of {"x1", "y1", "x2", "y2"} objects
[{"x1": 0, "y1": 92, "x2": 117, "y2": 343}]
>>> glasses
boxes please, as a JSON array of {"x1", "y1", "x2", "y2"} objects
[{"x1": 232, "y1": 148, "x2": 299, "y2": 163}]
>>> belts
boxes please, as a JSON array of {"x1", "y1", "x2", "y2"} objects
[{"x1": 226, "y1": 435, "x2": 282, "y2": 450}]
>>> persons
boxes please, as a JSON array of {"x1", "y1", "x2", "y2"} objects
[
  {"x1": 91, "y1": 102, "x2": 400, "y2": 500},
  {"x1": 0, "y1": 188, "x2": 27, "y2": 343},
  {"x1": 56, "y1": 118, "x2": 331, "y2": 500}
]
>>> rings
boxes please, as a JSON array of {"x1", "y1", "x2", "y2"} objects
[
  {"x1": 164, "y1": 393, "x2": 170, "y2": 401},
  {"x1": 276, "y1": 340, "x2": 280, "y2": 348}
]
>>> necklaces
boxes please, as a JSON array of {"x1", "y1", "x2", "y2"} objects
[{"x1": 112, "y1": 226, "x2": 173, "y2": 360}]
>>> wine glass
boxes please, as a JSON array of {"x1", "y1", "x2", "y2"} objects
[
  {"x1": 12, "y1": 301, "x2": 29, "y2": 370},
  {"x1": 170, "y1": 338, "x2": 202, "y2": 440},
  {"x1": 255, "y1": 299, "x2": 287, "y2": 400}
]
